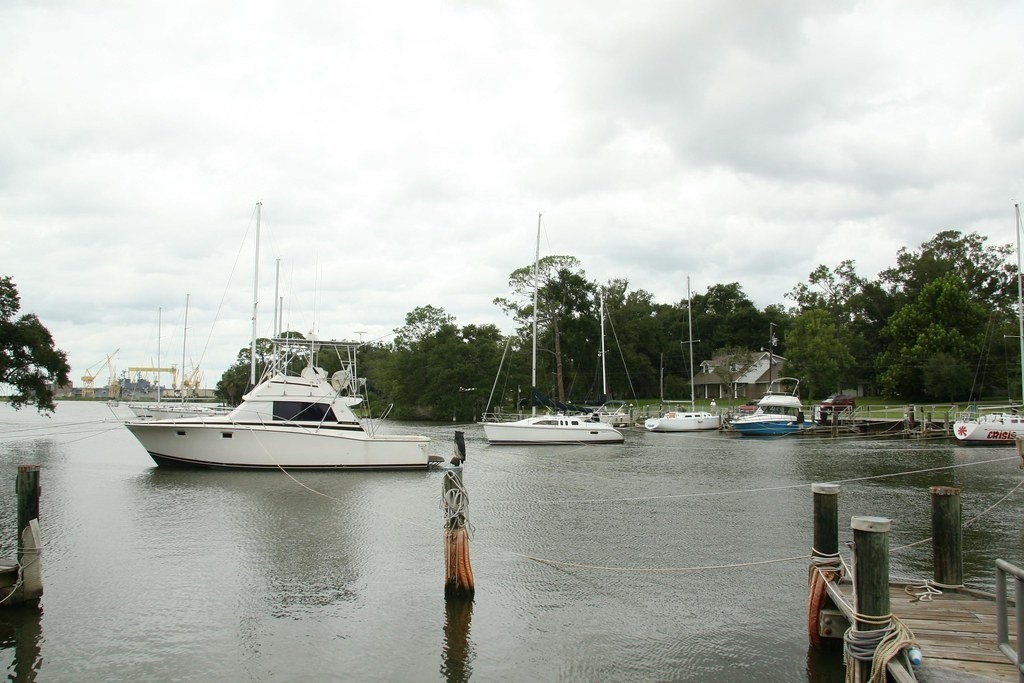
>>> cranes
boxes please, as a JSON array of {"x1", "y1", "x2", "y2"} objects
[{"x1": 80, "y1": 348, "x2": 121, "y2": 400}]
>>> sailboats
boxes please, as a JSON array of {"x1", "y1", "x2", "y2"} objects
[
  {"x1": 123, "y1": 206, "x2": 447, "y2": 477},
  {"x1": 641, "y1": 274, "x2": 727, "y2": 436},
  {"x1": 475, "y1": 210, "x2": 628, "y2": 446},
  {"x1": 584, "y1": 292, "x2": 633, "y2": 428},
  {"x1": 953, "y1": 203, "x2": 1022, "y2": 447}
]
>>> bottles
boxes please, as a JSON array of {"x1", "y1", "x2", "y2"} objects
[{"x1": 907, "y1": 647, "x2": 922, "y2": 664}]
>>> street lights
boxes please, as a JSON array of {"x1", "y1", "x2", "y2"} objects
[{"x1": 761, "y1": 323, "x2": 782, "y2": 388}]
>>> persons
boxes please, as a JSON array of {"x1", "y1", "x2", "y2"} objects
[{"x1": 710, "y1": 399, "x2": 716, "y2": 415}]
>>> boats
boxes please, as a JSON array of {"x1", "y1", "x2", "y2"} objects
[{"x1": 726, "y1": 376, "x2": 822, "y2": 438}]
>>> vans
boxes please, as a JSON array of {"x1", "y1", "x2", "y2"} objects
[{"x1": 819, "y1": 394, "x2": 856, "y2": 414}]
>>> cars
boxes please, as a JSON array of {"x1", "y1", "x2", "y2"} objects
[{"x1": 735, "y1": 400, "x2": 761, "y2": 411}]
